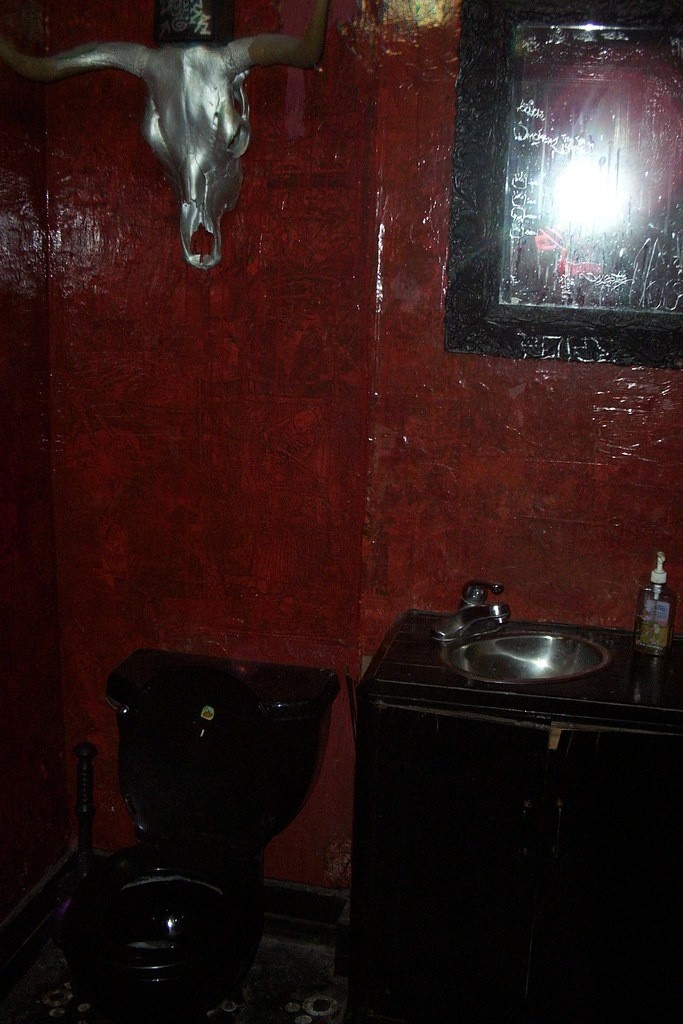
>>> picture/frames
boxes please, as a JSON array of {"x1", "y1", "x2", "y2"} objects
[{"x1": 444, "y1": 1, "x2": 683, "y2": 368}]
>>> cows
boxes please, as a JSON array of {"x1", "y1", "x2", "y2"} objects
[{"x1": 0, "y1": 0, "x2": 329, "y2": 270}]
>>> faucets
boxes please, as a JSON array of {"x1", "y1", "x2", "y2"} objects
[{"x1": 459, "y1": 577, "x2": 512, "y2": 628}]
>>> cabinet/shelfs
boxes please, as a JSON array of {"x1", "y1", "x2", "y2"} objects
[{"x1": 347, "y1": 694, "x2": 683, "y2": 1024}]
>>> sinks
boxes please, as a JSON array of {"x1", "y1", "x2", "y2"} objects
[{"x1": 440, "y1": 625, "x2": 615, "y2": 688}]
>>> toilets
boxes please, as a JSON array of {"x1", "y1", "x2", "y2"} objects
[{"x1": 59, "y1": 644, "x2": 342, "y2": 1024}]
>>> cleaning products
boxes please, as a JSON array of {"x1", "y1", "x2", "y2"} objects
[{"x1": 631, "y1": 551, "x2": 677, "y2": 656}]
